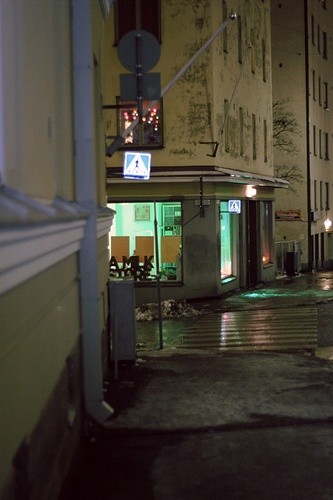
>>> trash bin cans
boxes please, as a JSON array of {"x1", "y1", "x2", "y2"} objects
[{"x1": 286, "y1": 251, "x2": 298, "y2": 276}]
